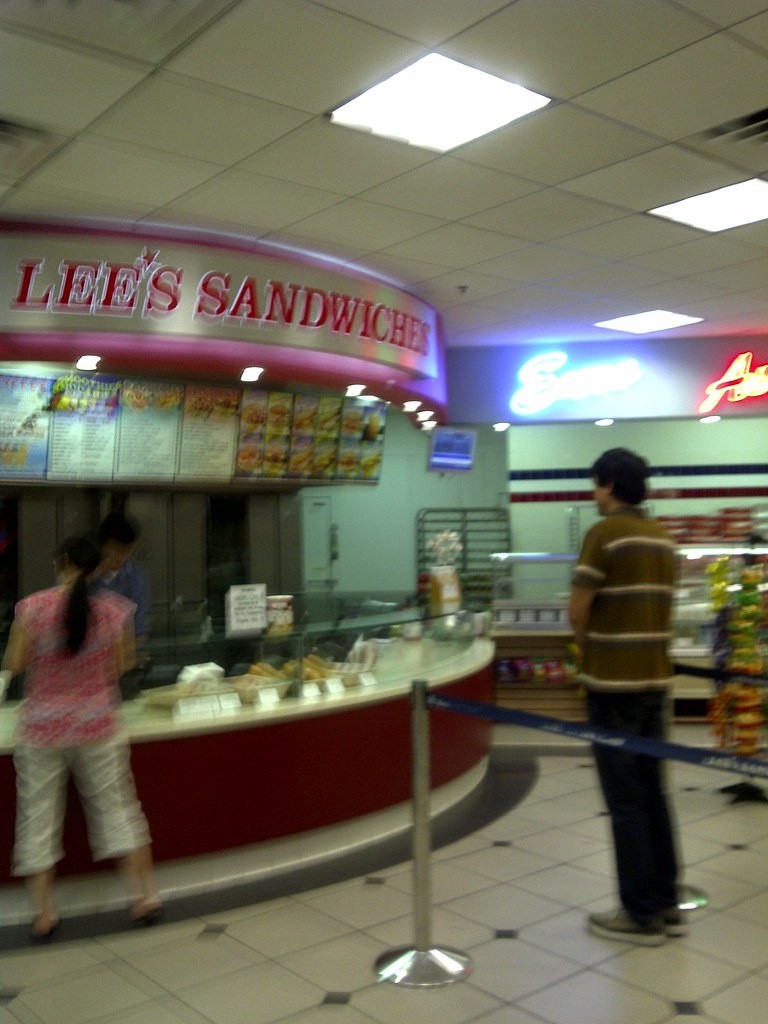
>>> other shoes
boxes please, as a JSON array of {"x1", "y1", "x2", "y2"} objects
[
  {"x1": 588, "y1": 906, "x2": 663, "y2": 946},
  {"x1": 663, "y1": 904, "x2": 691, "y2": 935},
  {"x1": 127, "y1": 905, "x2": 164, "y2": 929},
  {"x1": 30, "y1": 915, "x2": 61, "y2": 942}
]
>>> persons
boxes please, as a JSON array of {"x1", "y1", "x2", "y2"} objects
[
  {"x1": 89, "y1": 508, "x2": 150, "y2": 698},
  {"x1": 569, "y1": 447, "x2": 690, "y2": 945},
  {"x1": 4, "y1": 533, "x2": 163, "y2": 940}
]
